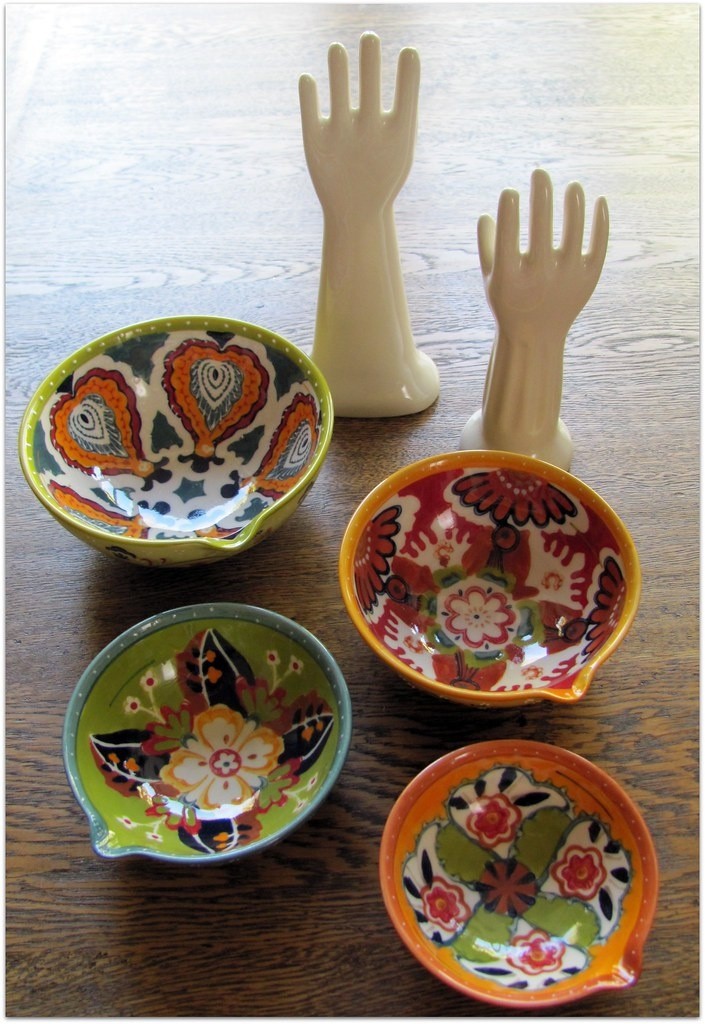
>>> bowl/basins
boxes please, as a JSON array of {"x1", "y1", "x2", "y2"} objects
[
  {"x1": 20, "y1": 312, "x2": 334, "y2": 570},
  {"x1": 378, "y1": 738, "x2": 661, "y2": 1008},
  {"x1": 61, "y1": 601, "x2": 353, "y2": 864},
  {"x1": 338, "y1": 450, "x2": 642, "y2": 708}
]
